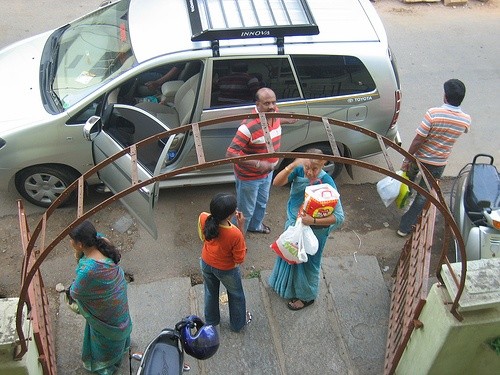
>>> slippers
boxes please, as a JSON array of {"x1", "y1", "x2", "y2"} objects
[{"x1": 287, "y1": 297, "x2": 317, "y2": 310}]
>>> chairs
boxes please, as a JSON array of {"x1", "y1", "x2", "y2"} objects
[
  {"x1": 139, "y1": 62, "x2": 195, "y2": 94},
  {"x1": 134, "y1": 73, "x2": 199, "y2": 149}
]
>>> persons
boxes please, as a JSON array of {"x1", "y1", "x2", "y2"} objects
[
  {"x1": 397, "y1": 79, "x2": 471, "y2": 236},
  {"x1": 137, "y1": 62, "x2": 185, "y2": 88},
  {"x1": 198, "y1": 192, "x2": 252, "y2": 331},
  {"x1": 268, "y1": 148, "x2": 345, "y2": 311},
  {"x1": 224, "y1": 87, "x2": 299, "y2": 235},
  {"x1": 65, "y1": 220, "x2": 132, "y2": 375}
]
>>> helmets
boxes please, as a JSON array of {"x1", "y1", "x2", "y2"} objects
[{"x1": 175, "y1": 313, "x2": 220, "y2": 361}]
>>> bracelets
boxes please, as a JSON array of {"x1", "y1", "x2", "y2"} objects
[
  {"x1": 403, "y1": 157, "x2": 410, "y2": 162},
  {"x1": 314, "y1": 217, "x2": 316, "y2": 225},
  {"x1": 255, "y1": 160, "x2": 261, "y2": 169},
  {"x1": 285, "y1": 166, "x2": 294, "y2": 173}
]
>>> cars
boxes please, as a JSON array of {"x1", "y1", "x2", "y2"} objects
[{"x1": 0, "y1": 1, "x2": 401, "y2": 241}]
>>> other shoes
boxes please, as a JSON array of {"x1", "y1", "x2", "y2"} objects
[
  {"x1": 257, "y1": 223, "x2": 271, "y2": 233},
  {"x1": 246, "y1": 311, "x2": 252, "y2": 325}
]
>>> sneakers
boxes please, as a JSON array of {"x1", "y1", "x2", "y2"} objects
[{"x1": 397, "y1": 228, "x2": 407, "y2": 237}]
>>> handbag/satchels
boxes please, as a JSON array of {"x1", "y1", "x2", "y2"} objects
[
  {"x1": 396, "y1": 171, "x2": 410, "y2": 210},
  {"x1": 269, "y1": 217, "x2": 319, "y2": 266},
  {"x1": 376, "y1": 169, "x2": 403, "y2": 208},
  {"x1": 298, "y1": 177, "x2": 340, "y2": 218}
]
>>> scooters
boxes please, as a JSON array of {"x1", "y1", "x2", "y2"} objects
[
  {"x1": 133, "y1": 326, "x2": 191, "y2": 375},
  {"x1": 450, "y1": 154, "x2": 500, "y2": 262}
]
